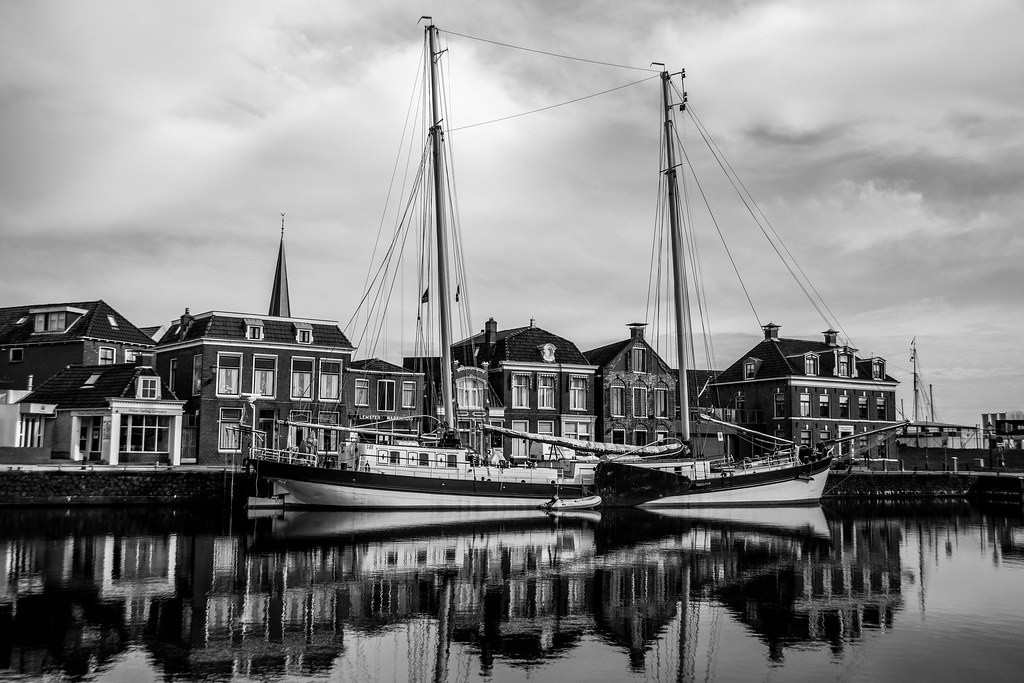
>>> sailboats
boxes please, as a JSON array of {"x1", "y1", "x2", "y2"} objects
[
  {"x1": 245, "y1": 15, "x2": 602, "y2": 511},
  {"x1": 598, "y1": 502, "x2": 834, "y2": 683},
  {"x1": 246, "y1": 493, "x2": 602, "y2": 683},
  {"x1": 599, "y1": 62, "x2": 910, "y2": 507}
]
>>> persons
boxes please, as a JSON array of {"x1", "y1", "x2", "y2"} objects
[{"x1": 245, "y1": 442, "x2": 252, "y2": 463}]
